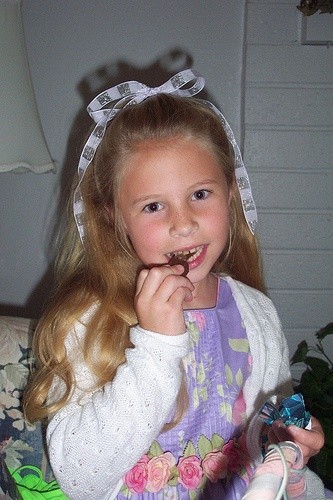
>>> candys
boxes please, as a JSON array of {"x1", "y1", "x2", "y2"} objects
[{"x1": 168, "y1": 256, "x2": 189, "y2": 277}]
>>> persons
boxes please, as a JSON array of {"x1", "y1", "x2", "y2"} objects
[{"x1": 23, "y1": 68, "x2": 333, "y2": 500}]
